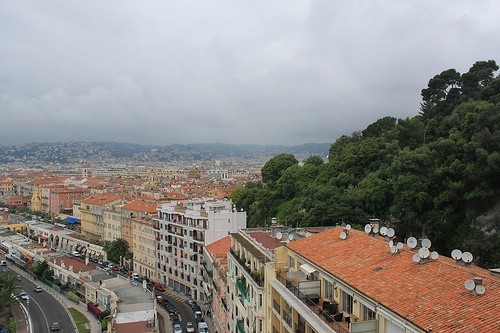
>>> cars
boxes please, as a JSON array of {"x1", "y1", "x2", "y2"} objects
[
  {"x1": 0, "y1": 260, "x2": 7, "y2": 266},
  {"x1": 0, "y1": 206, "x2": 82, "y2": 234},
  {"x1": 19, "y1": 292, "x2": 29, "y2": 300},
  {"x1": 16, "y1": 275, "x2": 21, "y2": 281},
  {"x1": 34, "y1": 285, "x2": 42, "y2": 293},
  {"x1": 51, "y1": 322, "x2": 59, "y2": 331},
  {"x1": 1, "y1": 267, "x2": 9, "y2": 273},
  {"x1": 73, "y1": 249, "x2": 210, "y2": 333}
]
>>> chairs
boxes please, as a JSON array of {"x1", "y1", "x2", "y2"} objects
[{"x1": 317, "y1": 307, "x2": 351, "y2": 323}]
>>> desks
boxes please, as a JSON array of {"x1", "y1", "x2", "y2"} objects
[
  {"x1": 305, "y1": 293, "x2": 320, "y2": 305},
  {"x1": 323, "y1": 299, "x2": 339, "y2": 315}
]
